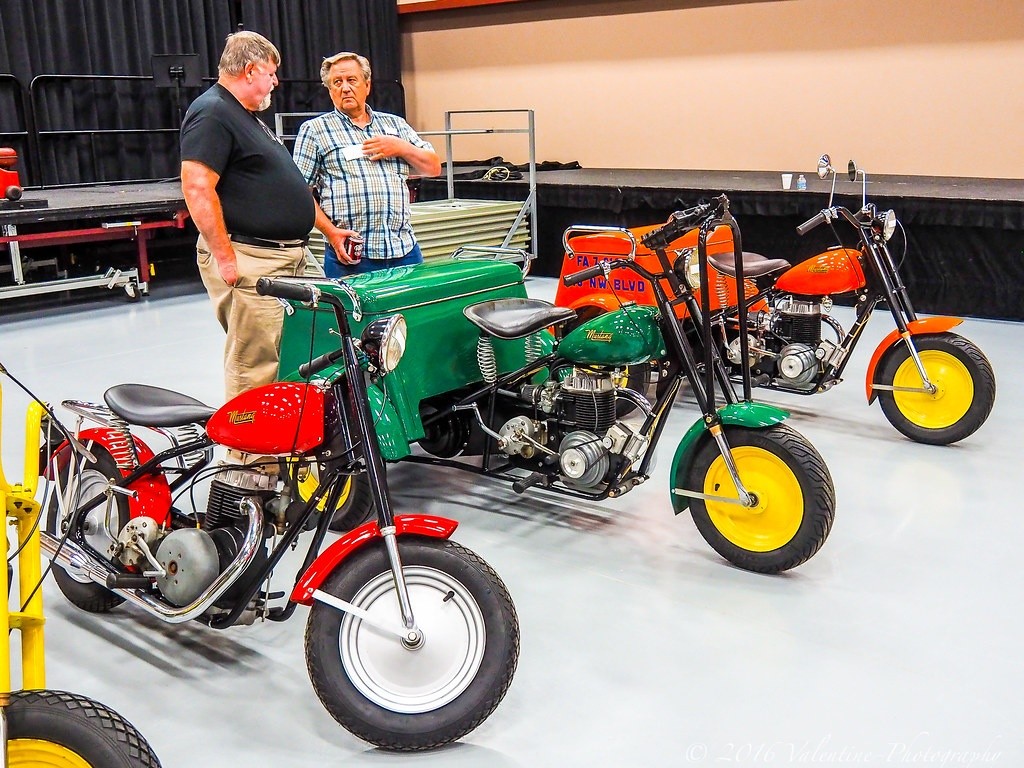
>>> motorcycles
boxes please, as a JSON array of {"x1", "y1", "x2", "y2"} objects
[
  {"x1": 0, "y1": 362, "x2": 164, "y2": 768},
  {"x1": 40, "y1": 275, "x2": 524, "y2": 759},
  {"x1": 547, "y1": 154, "x2": 998, "y2": 452},
  {"x1": 273, "y1": 194, "x2": 840, "y2": 578}
]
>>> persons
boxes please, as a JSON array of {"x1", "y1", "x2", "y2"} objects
[
  {"x1": 180, "y1": 31, "x2": 326, "y2": 481},
  {"x1": 293, "y1": 52, "x2": 442, "y2": 279}
]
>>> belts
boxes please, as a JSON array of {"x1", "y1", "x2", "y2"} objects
[{"x1": 231, "y1": 234, "x2": 305, "y2": 248}]
